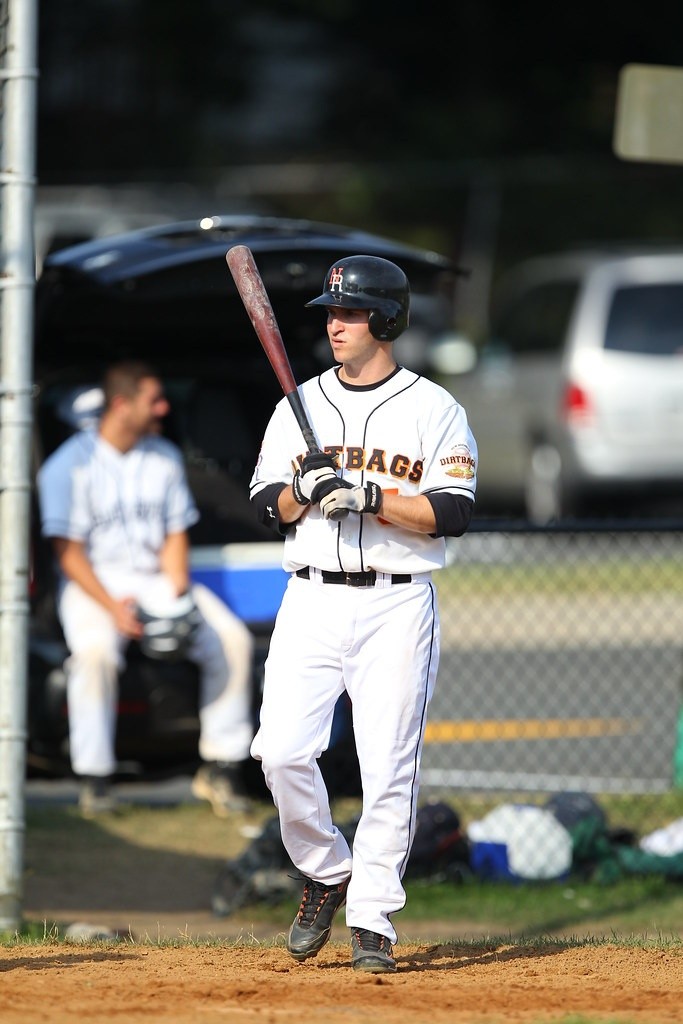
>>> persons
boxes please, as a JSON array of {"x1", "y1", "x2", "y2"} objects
[
  {"x1": 243, "y1": 251, "x2": 485, "y2": 979},
  {"x1": 33, "y1": 361, "x2": 259, "y2": 823}
]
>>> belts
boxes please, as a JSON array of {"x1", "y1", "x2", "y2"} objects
[{"x1": 297, "y1": 565, "x2": 411, "y2": 589}]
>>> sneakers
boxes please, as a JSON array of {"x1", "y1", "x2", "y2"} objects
[
  {"x1": 286, "y1": 871, "x2": 352, "y2": 962},
  {"x1": 349, "y1": 926, "x2": 399, "y2": 972}
]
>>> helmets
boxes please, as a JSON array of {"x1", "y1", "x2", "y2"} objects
[{"x1": 304, "y1": 255, "x2": 411, "y2": 342}]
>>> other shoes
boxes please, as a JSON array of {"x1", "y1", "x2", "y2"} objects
[
  {"x1": 78, "y1": 774, "x2": 112, "y2": 819},
  {"x1": 192, "y1": 761, "x2": 255, "y2": 817}
]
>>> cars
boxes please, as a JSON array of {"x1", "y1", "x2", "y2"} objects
[
  {"x1": 37, "y1": 211, "x2": 451, "y2": 521},
  {"x1": 460, "y1": 245, "x2": 683, "y2": 527}
]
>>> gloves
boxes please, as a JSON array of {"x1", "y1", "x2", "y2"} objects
[
  {"x1": 291, "y1": 451, "x2": 340, "y2": 506},
  {"x1": 310, "y1": 476, "x2": 383, "y2": 520}
]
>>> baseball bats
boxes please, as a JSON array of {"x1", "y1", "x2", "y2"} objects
[{"x1": 226, "y1": 245, "x2": 350, "y2": 522}]
